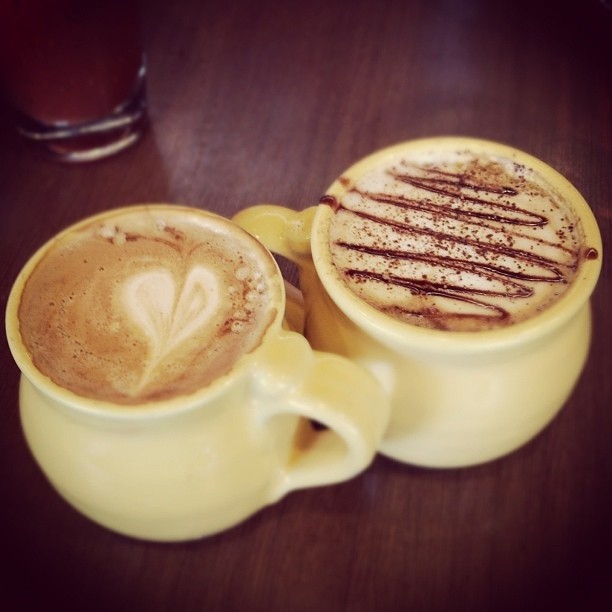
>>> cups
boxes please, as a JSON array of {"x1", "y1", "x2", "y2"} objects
[
  {"x1": 0, "y1": 0, "x2": 151, "y2": 165},
  {"x1": 233, "y1": 136, "x2": 603, "y2": 468},
  {"x1": 8, "y1": 205, "x2": 389, "y2": 540}
]
